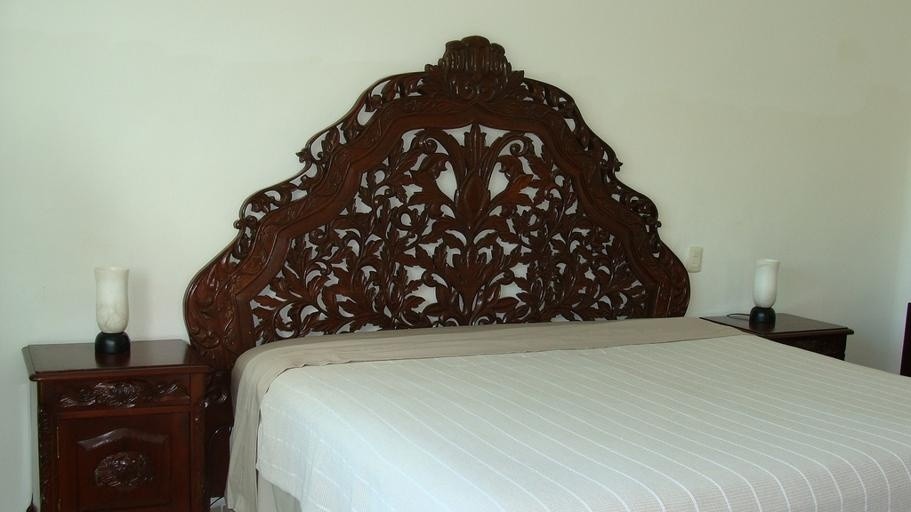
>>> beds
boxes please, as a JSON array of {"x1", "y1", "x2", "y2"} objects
[{"x1": 183, "y1": 37, "x2": 910, "y2": 510}]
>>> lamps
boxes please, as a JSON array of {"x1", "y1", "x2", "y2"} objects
[
  {"x1": 750, "y1": 258, "x2": 781, "y2": 328},
  {"x1": 93, "y1": 266, "x2": 130, "y2": 358}
]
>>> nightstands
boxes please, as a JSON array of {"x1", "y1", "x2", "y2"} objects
[
  {"x1": 699, "y1": 312, "x2": 855, "y2": 363},
  {"x1": 20, "y1": 339, "x2": 208, "y2": 511}
]
